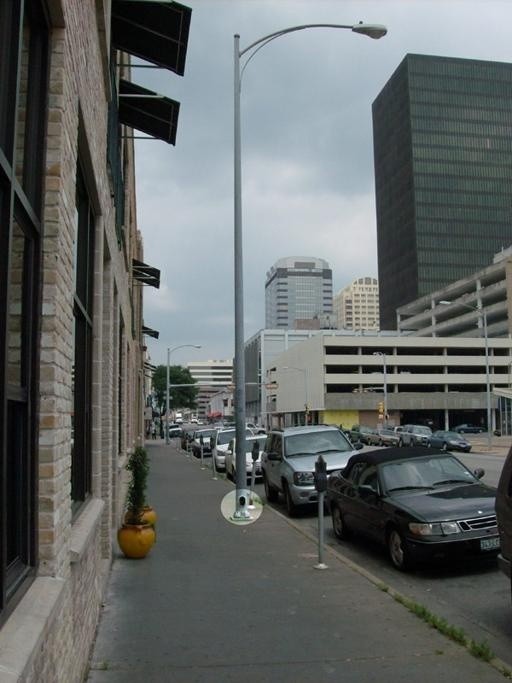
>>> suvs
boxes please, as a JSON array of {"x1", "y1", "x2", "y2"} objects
[
  {"x1": 260, "y1": 423, "x2": 363, "y2": 514},
  {"x1": 488, "y1": 442, "x2": 512, "y2": 580}
]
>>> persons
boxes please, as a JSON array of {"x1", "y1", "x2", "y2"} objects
[{"x1": 152, "y1": 422, "x2": 156, "y2": 440}]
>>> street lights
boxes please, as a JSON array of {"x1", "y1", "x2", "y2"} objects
[
  {"x1": 165, "y1": 339, "x2": 204, "y2": 449},
  {"x1": 438, "y1": 297, "x2": 495, "y2": 452},
  {"x1": 371, "y1": 350, "x2": 389, "y2": 426},
  {"x1": 232, "y1": 13, "x2": 385, "y2": 525},
  {"x1": 280, "y1": 365, "x2": 311, "y2": 425}
]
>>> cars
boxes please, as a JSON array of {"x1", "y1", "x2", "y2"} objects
[
  {"x1": 324, "y1": 443, "x2": 505, "y2": 571},
  {"x1": 452, "y1": 422, "x2": 485, "y2": 432},
  {"x1": 340, "y1": 419, "x2": 477, "y2": 455},
  {"x1": 222, "y1": 434, "x2": 267, "y2": 482},
  {"x1": 168, "y1": 409, "x2": 263, "y2": 456}
]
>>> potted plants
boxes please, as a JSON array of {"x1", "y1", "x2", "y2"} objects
[{"x1": 117, "y1": 445, "x2": 157, "y2": 559}]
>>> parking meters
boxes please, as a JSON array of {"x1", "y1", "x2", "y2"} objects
[{"x1": 309, "y1": 453, "x2": 334, "y2": 569}]
